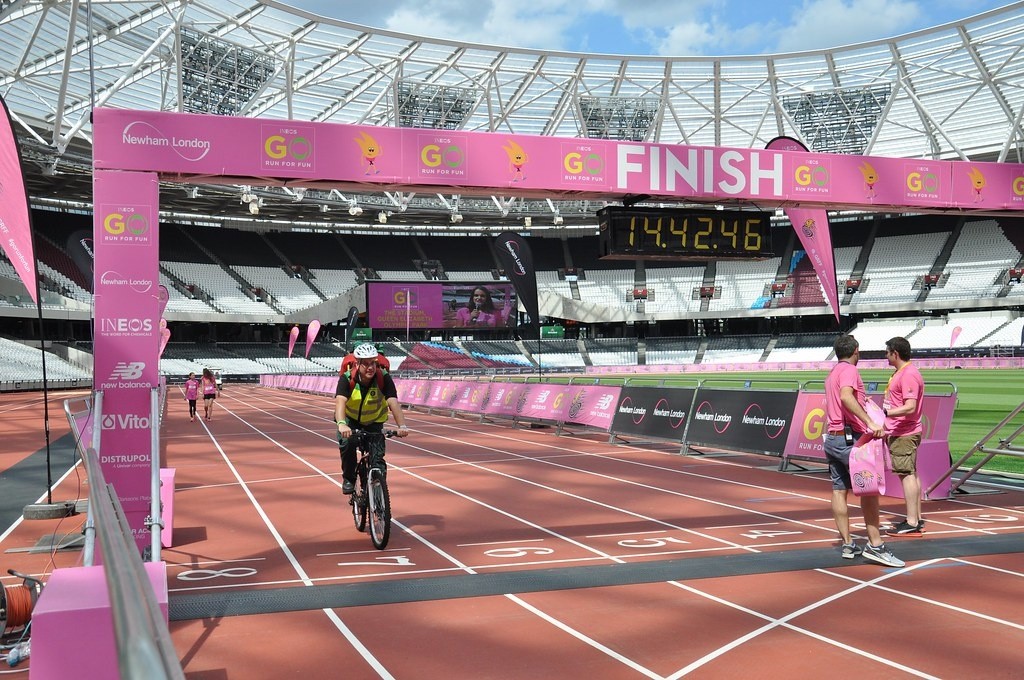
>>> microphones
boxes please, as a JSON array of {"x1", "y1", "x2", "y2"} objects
[{"x1": 472, "y1": 303, "x2": 479, "y2": 324}]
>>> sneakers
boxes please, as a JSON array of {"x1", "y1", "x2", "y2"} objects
[
  {"x1": 841, "y1": 540, "x2": 862, "y2": 559},
  {"x1": 342, "y1": 478, "x2": 354, "y2": 495},
  {"x1": 885, "y1": 524, "x2": 923, "y2": 536},
  {"x1": 891, "y1": 519, "x2": 926, "y2": 532},
  {"x1": 862, "y1": 540, "x2": 905, "y2": 567}
]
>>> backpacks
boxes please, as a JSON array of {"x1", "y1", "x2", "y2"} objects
[{"x1": 338, "y1": 353, "x2": 390, "y2": 381}]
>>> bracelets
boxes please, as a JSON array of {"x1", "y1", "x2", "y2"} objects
[
  {"x1": 399, "y1": 424, "x2": 406, "y2": 429},
  {"x1": 337, "y1": 420, "x2": 347, "y2": 426}
]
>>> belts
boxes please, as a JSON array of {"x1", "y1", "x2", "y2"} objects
[{"x1": 829, "y1": 430, "x2": 845, "y2": 436}]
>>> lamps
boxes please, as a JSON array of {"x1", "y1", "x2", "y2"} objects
[
  {"x1": 554, "y1": 217, "x2": 563, "y2": 225},
  {"x1": 241, "y1": 194, "x2": 258, "y2": 204},
  {"x1": 348, "y1": 207, "x2": 364, "y2": 217},
  {"x1": 452, "y1": 215, "x2": 464, "y2": 223}
]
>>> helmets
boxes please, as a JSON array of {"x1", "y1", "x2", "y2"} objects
[{"x1": 353, "y1": 343, "x2": 378, "y2": 359}]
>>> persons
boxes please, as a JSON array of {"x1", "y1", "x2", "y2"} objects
[
  {"x1": 184, "y1": 372, "x2": 199, "y2": 421},
  {"x1": 883, "y1": 408, "x2": 887, "y2": 417},
  {"x1": 456, "y1": 286, "x2": 503, "y2": 328},
  {"x1": 334, "y1": 344, "x2": 408, "y2": 521},
  {"x1": 823, "y1": 334, "x2": 905, "y2": 568},
  {"x1": 450, "y1": 298, "x2": 457, "y2": 310},
  {"x1": 216, "y1": 372, "x2": 219, "y2": 378},
  {"x1": 201, "y1": 368, "x2": 220, "y2": 422},
  {"x1": 882, "y1": 337, "x2": 927, "y2": 537}
]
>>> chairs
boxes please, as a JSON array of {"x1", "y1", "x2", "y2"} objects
[{"x1": 0, "y1": 231, "x2": 94, "y2": 384}]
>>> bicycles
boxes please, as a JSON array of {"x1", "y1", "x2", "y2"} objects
[{"x1": 339, "y1": 430, "x2": 398, "y2": 551}]
